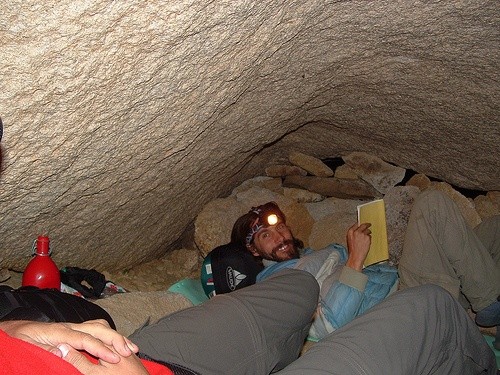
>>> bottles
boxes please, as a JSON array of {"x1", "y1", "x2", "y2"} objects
[{"x1": 22, "y1": 236, "x2": 61, "y2": 291}]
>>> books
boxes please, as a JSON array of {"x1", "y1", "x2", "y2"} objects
[{"x1": 357, "y1": 199, "x2": 390, "y2": 268}]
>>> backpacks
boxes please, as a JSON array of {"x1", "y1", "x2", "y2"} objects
[
  {"x1": 0, "y1": 285, "x2": 116, "y2": 360},
  {"x1": 59, "y1": 266, "x2": 106, "y2": 300}
]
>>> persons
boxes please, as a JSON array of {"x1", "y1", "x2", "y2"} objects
[
  {"x1": 231, "y1": 190, "x2": 500, "y2": 343},
  {"x1": 0, "y1": 269, "x2": 500, "y2": 375}
]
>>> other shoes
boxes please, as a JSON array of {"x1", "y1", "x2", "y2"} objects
[{"x1": 476, "y1": 303, "x2": 500, "y2": 351}]
